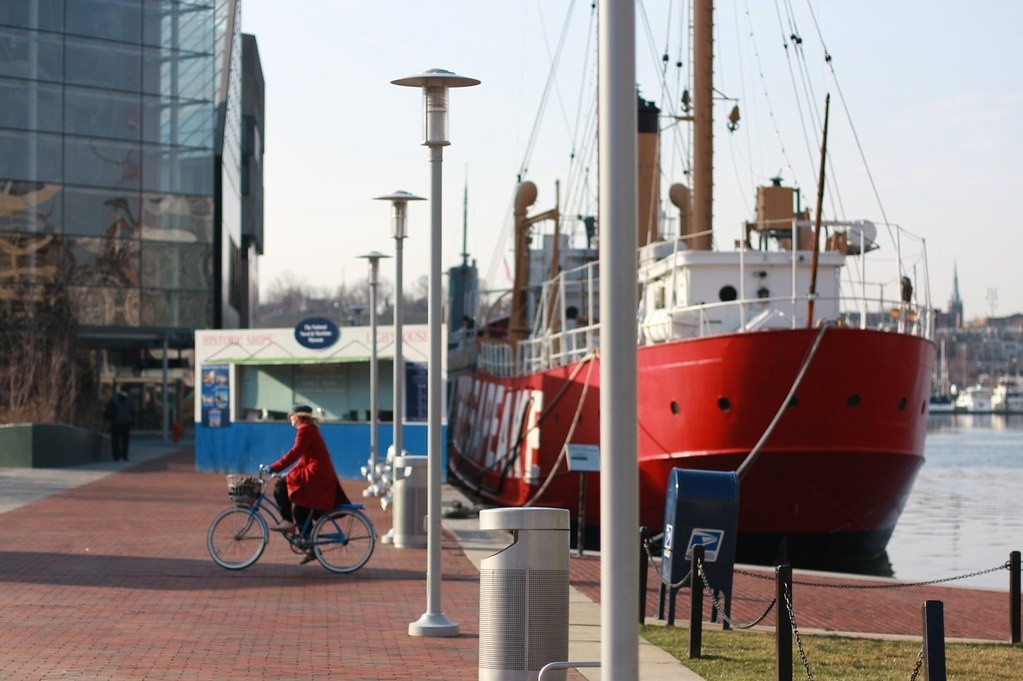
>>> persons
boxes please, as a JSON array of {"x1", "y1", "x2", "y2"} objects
[
  {"x1": 264, "y1": 406, "x2": 350, "y2": 565},
  {"x1": 103, "y1": 392, "x2": 135, "y2": 461}
]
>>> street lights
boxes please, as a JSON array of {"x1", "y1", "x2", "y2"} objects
[
  {"x1": 355, "y1": 250, "x2": 393, "y2": 478},
  {"x1": 362, "y1": 189, "x2": 427, "y2": 498},
  {"x1": 390, "y1": 68, "x2": 481, "y2": 636}
]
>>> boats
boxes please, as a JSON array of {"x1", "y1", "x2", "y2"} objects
[{"x1": 956, "y1": 374, "x2": 1023, "y2": 414}]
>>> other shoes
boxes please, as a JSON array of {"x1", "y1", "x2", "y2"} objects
[
  {"x1": 269, "y1": 520, "x2": 296, "y2": 533},
  {"x1": 298, "y1": 548, "x2": 322, "y2": 566}
]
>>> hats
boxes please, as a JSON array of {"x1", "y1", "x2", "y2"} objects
[{"x1": 291, "y1": 405, "x2": 313, "y2": 414}]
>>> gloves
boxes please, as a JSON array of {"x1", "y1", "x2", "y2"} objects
[{"x1": 265, "y1": 464, "x2": 274, "y2": 474}]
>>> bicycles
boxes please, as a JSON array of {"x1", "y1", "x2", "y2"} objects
[{"x1": 207, "y1": 464, "x2": 378, "y2": 573}]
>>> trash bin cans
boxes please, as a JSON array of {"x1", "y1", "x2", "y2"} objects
[
  {"x1": 392, "y1": 455, "x2": 428, "y2": 548},
  {"x1": 477, "y1": 506, "x2": 572, "y2": 681},
  {"x1": 659, "y1": 466, "x2": 740, "y2": 633}
]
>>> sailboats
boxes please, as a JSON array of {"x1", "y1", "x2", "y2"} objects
[
  {"x1": 928, "y1": 338, "x2": 955, "y2": 413},
  {"x1": 443, "y1": 0, "x2": 937, "y2": 566}
]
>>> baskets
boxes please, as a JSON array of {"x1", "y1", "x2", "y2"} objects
[{"x1": 226, "y1": 475, "x2": 259, "y2": 503}]
